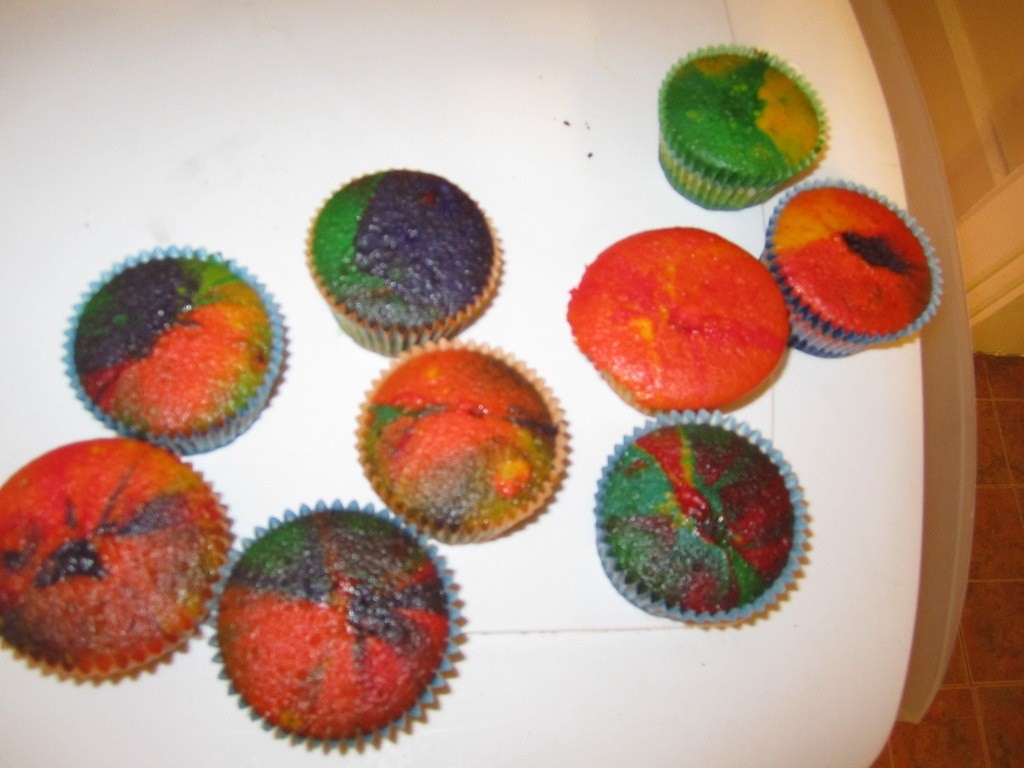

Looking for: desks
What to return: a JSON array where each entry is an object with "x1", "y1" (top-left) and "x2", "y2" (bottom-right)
[{"x1": 0, "y1": 1, "x2": 927, "y2": 768}]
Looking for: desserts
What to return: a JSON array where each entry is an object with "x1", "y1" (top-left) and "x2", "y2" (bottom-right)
[
  {"x1": 593, "y1": 407, "x2": 809, "y2": 624},
  {"x1": 567, "y1": 42, "x2": 947, "y2": 420},
  {"x1": 0, "y1": 169, "x2": 570, "y2": 750}
]
